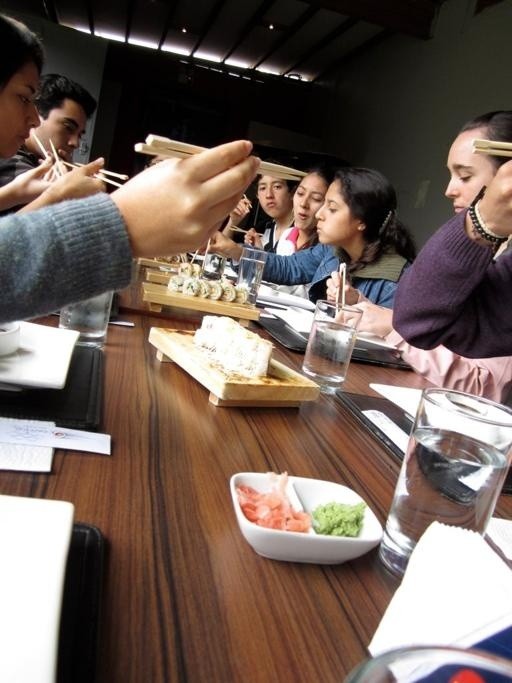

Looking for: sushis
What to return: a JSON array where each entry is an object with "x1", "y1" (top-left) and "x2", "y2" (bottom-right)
[{"x1": 166, "y1": 262, "x2": 249, "y2": 304}]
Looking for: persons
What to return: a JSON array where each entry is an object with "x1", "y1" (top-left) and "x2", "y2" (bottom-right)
[
  {"x1": 196, "y1": 109, "x2": 511, "y2": 407},
  {"x1": 0, "y1": 14, "x2": 263, "y2": 324}
]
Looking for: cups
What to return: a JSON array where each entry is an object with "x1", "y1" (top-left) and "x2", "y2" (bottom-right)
[
  {"x1": 378, "y1": 387, "x2": 512, "y2": 583},
  {"x1": 236, "y1": 247, "x2": 269, "y2": 306},
  {"x1": 298, "y1": 299, "x2": 364, "y2": 395},
  {"x1": 58, "y1": 290, "x2": 113, "y2": 346}
]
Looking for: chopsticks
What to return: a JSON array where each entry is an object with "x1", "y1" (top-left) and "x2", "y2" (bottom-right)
[
  {"x1": 32, "y1": 133, "x2": 310, "y2": 188},
  {"x1": 190, "y1": 238, "x2": 211, "y2": 271},
  {"x1": 472, "y1": 139, "x2": 512, "y2": 158},
  {"x1": 242, "y1": 192, "x2": 252, "y2": 209},
  {"x1": 228, "y1": 224, "x2": 266, "y2": 237},
  {"x1": 334, "y1": 263, "x2": 347, "y2": 320}
]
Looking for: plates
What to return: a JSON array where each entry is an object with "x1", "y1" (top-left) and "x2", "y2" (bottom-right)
[
  {"x1": 229, "y1": 471, "x2": 383, "y2": 566},
  {"x1": 239, "y1": 280, "x2": 400, "y2": 353},
  {"x1": 0, "y1": 321, "x2": 80, "y2": 390}
]
